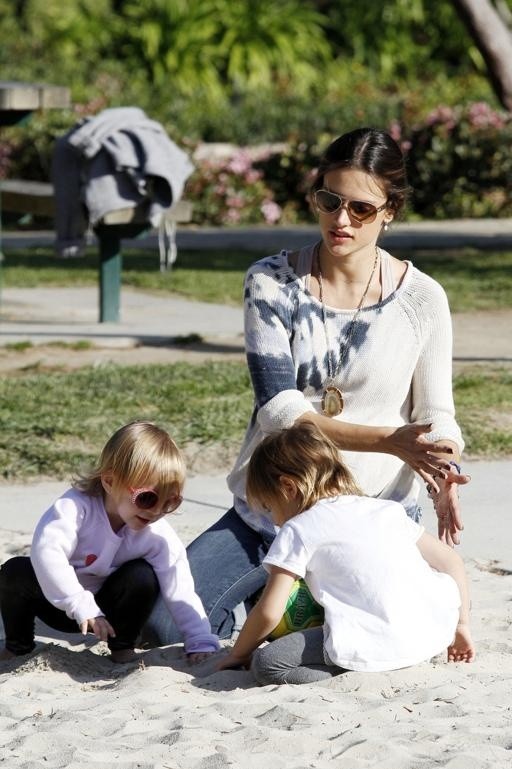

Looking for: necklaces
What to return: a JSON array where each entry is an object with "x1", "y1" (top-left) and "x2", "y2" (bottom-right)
[{"x1": 316, "y1": 236, "x2": 378, "y2": 417}]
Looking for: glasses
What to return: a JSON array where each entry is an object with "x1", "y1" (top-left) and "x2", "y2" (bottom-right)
[
  {"x1": 126, "y1": 481, "x2": 186, "y2": 516},
  {"x1": 308, "y1": 186, "x2": 393, "y2": 227}
]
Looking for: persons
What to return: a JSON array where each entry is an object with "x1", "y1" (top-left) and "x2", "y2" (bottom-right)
[
  {"x1": 139, "y1": 126, "x2": 472, "y2": 648},
  {"x1": 195, "y1": 418, "x2": 476, "y2": 686},
  {"x1": 1, "y1": 420, "x2": 221, "y2": 666}
]
